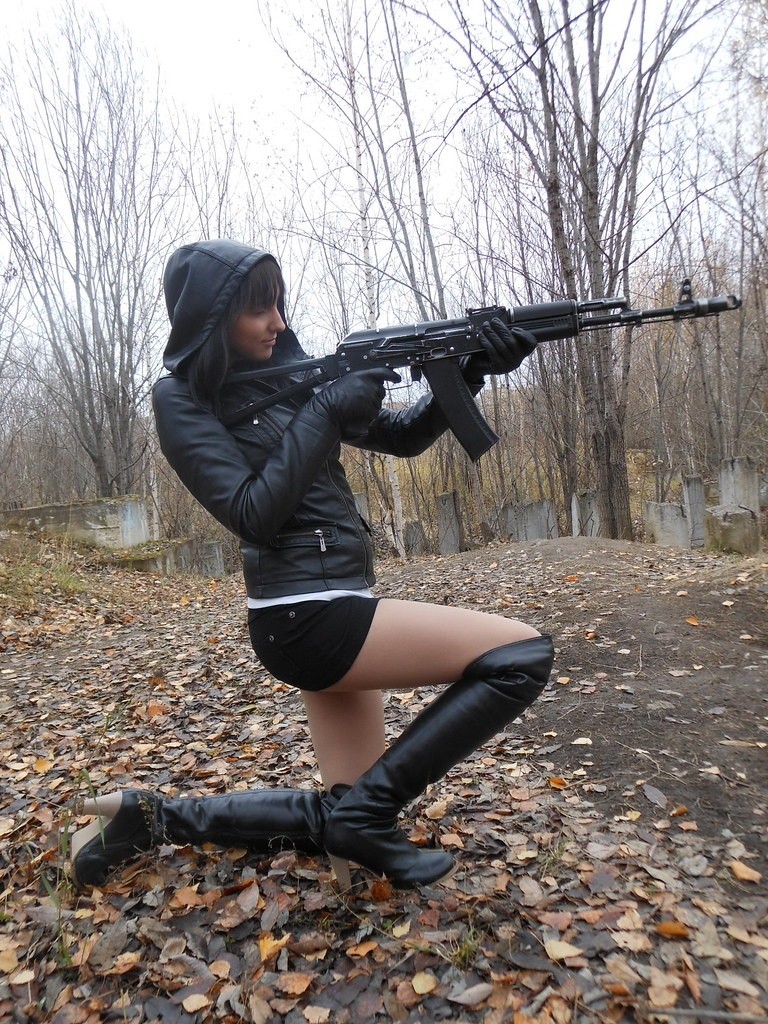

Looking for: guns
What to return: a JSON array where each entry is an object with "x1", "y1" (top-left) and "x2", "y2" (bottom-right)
[{"x1": 213, "y1": 278, "x2": 746, "y2": 461}]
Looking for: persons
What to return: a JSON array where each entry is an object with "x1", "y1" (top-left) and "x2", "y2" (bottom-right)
[{"x1": 72, "y1": 238, "x2": 555, "y2": 894}]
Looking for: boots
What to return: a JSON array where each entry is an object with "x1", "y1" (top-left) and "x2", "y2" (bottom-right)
[
  {"x1": 324, "y1": 632, "x2": 555, "y2": 894},
  {"x1": 70, "y1": 782, "x2": 354, "y2": 893}
]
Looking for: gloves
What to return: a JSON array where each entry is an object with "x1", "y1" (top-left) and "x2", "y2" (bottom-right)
[
  {"x1": 464, "y1": 317, "x2": 537, "y2": 383},
  {"x1": 304, "y1": 367, "x2": 401, "y2": 428}
]
